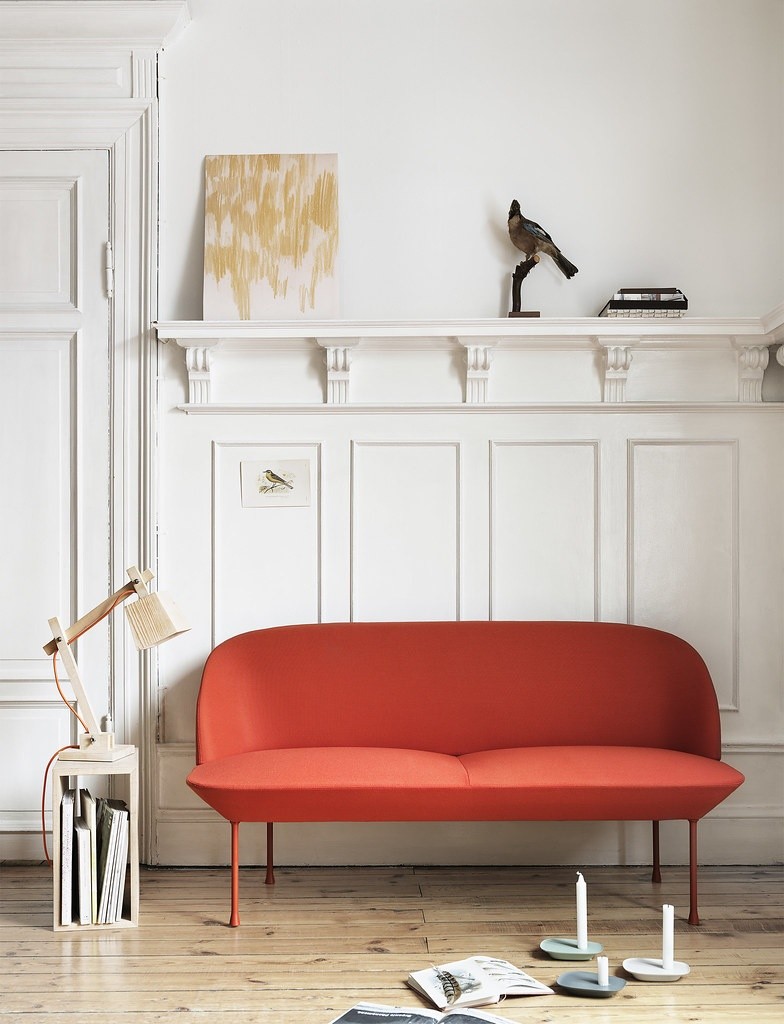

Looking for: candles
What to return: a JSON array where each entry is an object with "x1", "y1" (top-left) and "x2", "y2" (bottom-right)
[
  {"x1": 575, "y1": 872, "x2": 588, "y2": 949},
  {"x1": 662, "y1": 903, "x2": 675, "y2": 970},
  {"x1": 597, "y1": 956, "x2": 610, "y2": 986}
]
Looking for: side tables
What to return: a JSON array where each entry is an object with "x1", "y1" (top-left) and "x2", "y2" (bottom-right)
[{"x1": 51, "y1": 748, "x2": 142, "y2": 933}]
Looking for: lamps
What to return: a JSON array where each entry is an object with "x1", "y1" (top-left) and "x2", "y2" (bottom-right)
[{"x1": 42, "y1": 565, "x2": 192, "y2": 763}]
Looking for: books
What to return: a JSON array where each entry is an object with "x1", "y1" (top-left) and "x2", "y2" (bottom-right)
[
  {"x1": 404, "y1": 955, "x2": 556, "y2": 1012},
  {"x1": 328, "y1": 1001, "x2": 518, "y2": 1024},
  {"x1": 59, "y1": 789, "x2": 130, "y2": 926},
  {"x1": 598, "y1": 287, "x2": 688, "y2": 318}
]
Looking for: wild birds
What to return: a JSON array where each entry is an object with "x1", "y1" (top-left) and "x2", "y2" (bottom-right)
[{"x1": 507, "y1": 199, "x2": 578, "y2": 280}]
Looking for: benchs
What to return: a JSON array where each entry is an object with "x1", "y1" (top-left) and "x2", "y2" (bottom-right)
[{"x1": 185, "y1": 621, "x2": 744, "y2": 926}]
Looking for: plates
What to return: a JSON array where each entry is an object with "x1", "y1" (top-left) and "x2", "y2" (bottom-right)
[
  {"x1": 540, "y1": 939, "x2": 603, "y2": 961},
  {"x1": 557, "y1": 971, "x2": 626, "y2": 998},
  {"x1": 622, "y1": 957, "x2": 691, "y2": 982}
]
